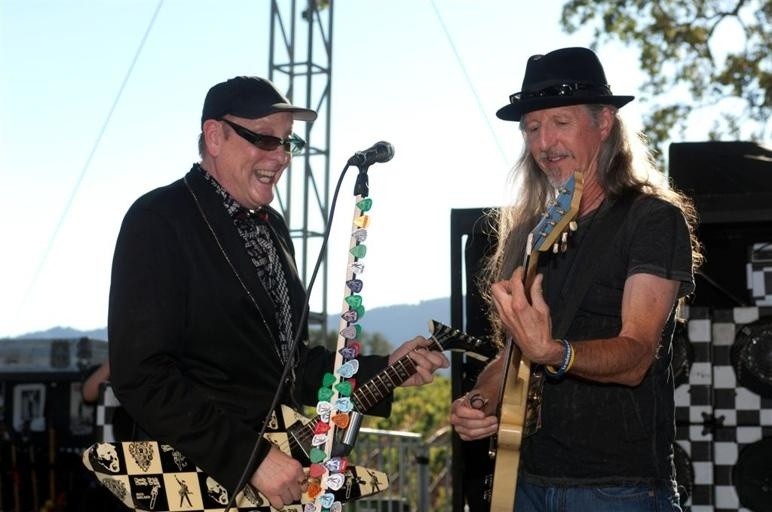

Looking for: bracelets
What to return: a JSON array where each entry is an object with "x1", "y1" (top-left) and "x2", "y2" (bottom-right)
[{"x1": 545, "y1": 335, "x2": 574, "y2": 377}]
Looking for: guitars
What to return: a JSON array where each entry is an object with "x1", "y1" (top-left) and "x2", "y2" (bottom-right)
[
  {"x1": 81, "y1": 320, "x2": 500, "y2": 510},
  {"x1": 462, "y1": 170, "x2": 584, "y2": 511}
]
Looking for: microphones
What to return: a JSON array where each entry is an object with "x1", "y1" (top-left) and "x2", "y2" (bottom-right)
[{"x1": 347, "y1": 141, "x2": 393, "y2": 166}]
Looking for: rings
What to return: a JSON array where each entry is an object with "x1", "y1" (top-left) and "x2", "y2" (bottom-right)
[{"x1": 298, "y1": 477, "x2": 308, "y2": 485}]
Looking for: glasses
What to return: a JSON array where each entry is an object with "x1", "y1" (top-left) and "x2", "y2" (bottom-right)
[
  {"x1": 509, "y1": 80, "x2": 606, "y2": 105},
  {"x1": 221, "y1": 118, "x2": 306, "y2": 156}
]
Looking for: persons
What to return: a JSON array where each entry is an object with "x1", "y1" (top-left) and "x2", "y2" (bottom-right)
[
  {"x1": 174, "y1": 475, "x2": 193, "y2": 508},
  {"x1": 82, "y1": 361, "x2": 112, "y2": 406},
  {"x1": 366, "y1": 470, "x2": 381, "y2": 494},
  {"x1": 450, "y1": 48, "x2": 702, "y2": 511},
  {"x1": 106, "y1": 76, "x2": 450, "y2": 511}
]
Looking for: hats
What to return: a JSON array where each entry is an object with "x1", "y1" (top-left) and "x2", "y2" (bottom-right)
[
  {"x1": 495, "y1": 46, "x2": 635, "y2": 123},
  {"x1": 200, "y1": 75, "x2": 318, "y2": 122}
]
